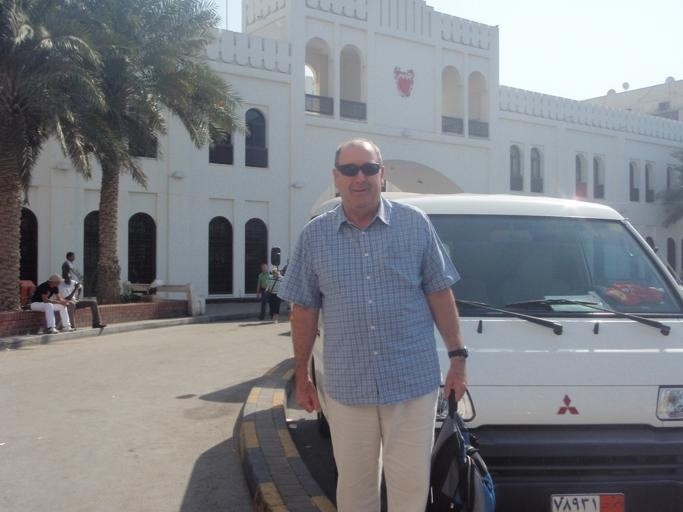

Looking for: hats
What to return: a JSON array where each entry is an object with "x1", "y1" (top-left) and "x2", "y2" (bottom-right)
[{"x1": 47, "y1": 274, "x2": 65, "y2": 282}]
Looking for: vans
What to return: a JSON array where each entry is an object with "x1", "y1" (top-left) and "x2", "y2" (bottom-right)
[{"x1": 305, "y1": 188, "x2": 683, "y2": 511}]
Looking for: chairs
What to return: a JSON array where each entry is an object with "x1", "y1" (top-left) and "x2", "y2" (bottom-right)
[{"x1": 499, "y1": 249, "x2": 573, "y2": 303}]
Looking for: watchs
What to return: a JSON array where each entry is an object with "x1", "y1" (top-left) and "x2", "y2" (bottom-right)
[{"x1": 446, "y1": 348, "x2": 468, "y2": 359}]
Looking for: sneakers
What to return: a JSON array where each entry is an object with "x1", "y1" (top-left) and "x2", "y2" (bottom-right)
[{"x1": 47, "y1": 327, "x2": 76, "y2": 333}]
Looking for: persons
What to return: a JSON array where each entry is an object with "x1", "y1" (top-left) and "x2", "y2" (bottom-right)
[
  {"x1": 276, "y1": 138, "x2": 469, "y2": 512},
  {"x1": 28, "y1": 251, "x2": 107, "y2": 335},
  {"x1": 256, "y1": 262, "x2": 282, "y2": 322}
]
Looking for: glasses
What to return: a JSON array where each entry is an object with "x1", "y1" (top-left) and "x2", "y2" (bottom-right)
[{"x1": 336, "y1": 163, "x2": 381, "y2": 176}]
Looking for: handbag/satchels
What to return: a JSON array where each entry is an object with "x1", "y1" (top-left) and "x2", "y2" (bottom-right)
[{"x1": 265, "y1": 294, "x2": 272, "y2": 302}]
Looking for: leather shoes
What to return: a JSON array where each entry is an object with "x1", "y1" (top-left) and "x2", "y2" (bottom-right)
[{"x1": 93, "y1": 323, "x2": 106, "y2": 328}]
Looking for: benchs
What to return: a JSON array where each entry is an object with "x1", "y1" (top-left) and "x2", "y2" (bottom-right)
[{"x1": 125, "y1": 281, "x2": 206, "y2": 317}]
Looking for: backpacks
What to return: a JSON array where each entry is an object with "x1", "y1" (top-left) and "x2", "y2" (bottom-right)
[{"x1": 425, "y1": 389, "x2": 496, "y2": 512}]
